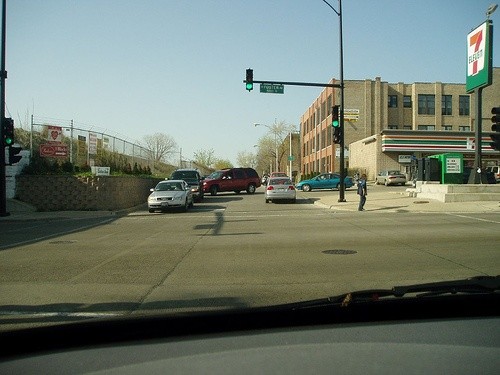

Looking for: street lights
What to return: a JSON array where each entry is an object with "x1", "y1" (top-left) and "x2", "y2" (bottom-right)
[
  {"x1": 254, "y1": 144, "x2": 273, "y2": 176},
  {"x1": 255, "y1": 123, "x2": 278, "y2": 173}
]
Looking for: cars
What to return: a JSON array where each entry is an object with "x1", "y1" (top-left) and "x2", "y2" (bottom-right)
[
  {"x1": 147, "y1": 180, "x2": 194, "y2": 213},
  {"x1": 264, "y1": 176, "x2": 297, "y2": 203},
  {"x1": 266, "y1": 172, "x2": 289, "y2": 186},
  {"x1": 295, "y1": 173, "x2": 356, "y2": 191},
  {"x1": 376, "y1": 169, "x2": 407, "y2": 186}
]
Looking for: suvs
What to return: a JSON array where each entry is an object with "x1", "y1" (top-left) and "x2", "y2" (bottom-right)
[{"x1": 164, "y1": 169, "x2": 205, "y2": 203}]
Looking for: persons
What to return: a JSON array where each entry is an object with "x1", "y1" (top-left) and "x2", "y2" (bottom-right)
[{"x1": 357, "y1": 174, "x2": 367, "y2": 211}]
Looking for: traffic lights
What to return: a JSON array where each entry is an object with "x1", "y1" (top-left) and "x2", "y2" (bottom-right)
[
  {"x1": 489, "y1": 106, "x2": 500, "y2": 151},
  {"x1": 4, "y1": 117, "x2": 15, "y2": 146},
  {"x1": 331, "y1": 104, "x2": 339, "y2": 127},
  {"x1": 9, "y1": 146, "x2": 23, "y2": 162},
  {"x1": 245, "y1": 68, "x2": 253, "y2": 90},
  {"x1": 333, "y1": 127, "x2": 341, "y2": 143}
]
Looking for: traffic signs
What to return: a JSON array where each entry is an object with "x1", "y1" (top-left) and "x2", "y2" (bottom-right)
[{"x1": 40, "y1": 143, "x2": 68, "y2": 155}]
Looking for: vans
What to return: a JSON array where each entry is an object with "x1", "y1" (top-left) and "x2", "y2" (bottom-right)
[
  {"x1": 483, "y1": 166, "x2": 500, "y2": 183},
  {"x1": 202, "y1": 168, "x2": 261, "y2": 196}
]
